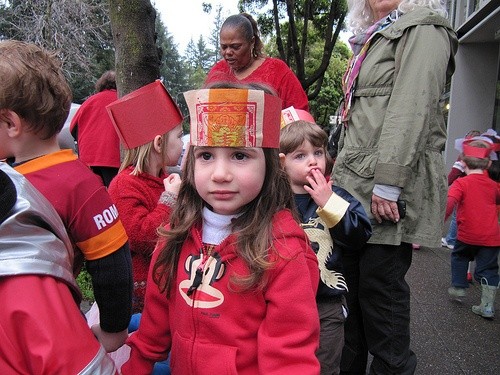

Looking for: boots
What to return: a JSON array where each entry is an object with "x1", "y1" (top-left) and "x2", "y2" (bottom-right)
[
  {"x1": 448, "y1": 286, "x2": 466, "y2": 302},
  {"x1": 471, "y1": 277, "x2": 500, "y2": 318}
]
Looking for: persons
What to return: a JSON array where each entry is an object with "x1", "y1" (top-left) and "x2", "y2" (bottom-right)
[
  {"x1": 0, "y1": 35, "x2": 500, "y2": 375},
  {"x1": 328, "y1": 0, "x2": 457, "y2": 375},
  {"x1": 200, "y1": 14, "x2": 310, "y2": 115}
]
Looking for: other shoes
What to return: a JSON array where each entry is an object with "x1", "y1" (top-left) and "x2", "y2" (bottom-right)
[{"x1": 441, "y1": 238, "x2": 454, "y2": 249}]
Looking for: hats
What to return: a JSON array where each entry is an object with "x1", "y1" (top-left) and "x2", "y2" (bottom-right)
[
  {"x1": 105, "y1": 80, "x2": 183, "y2": 150},
  {"x1": 281, "y1": 106, "x2": 317, "y2": 130}
]
